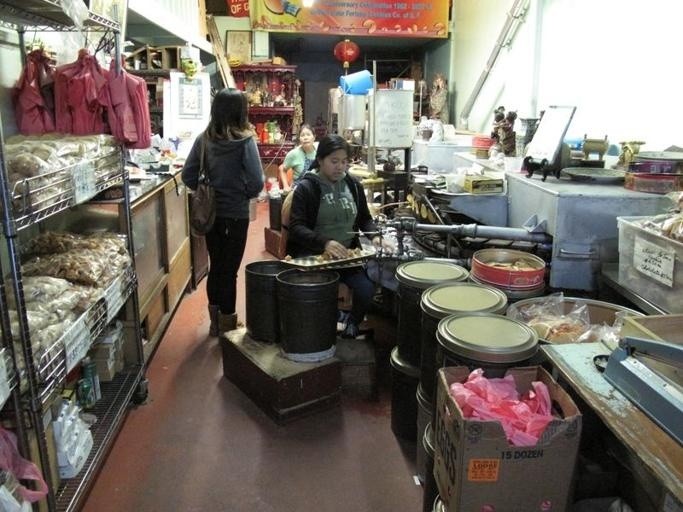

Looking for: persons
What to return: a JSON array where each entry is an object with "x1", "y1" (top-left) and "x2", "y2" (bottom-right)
[
  {"x1": 429, "y1": 74, "x2": 450, "y2": 123},
  {"x1": 278, "y1": 124, "x2": 319, "y2": 192},
  {"x1": 284, "y1": 134, "x2": 396, "y2": 340},
  {"x1": 181, "y1": 87, "x2": 263, "y2": 339}
]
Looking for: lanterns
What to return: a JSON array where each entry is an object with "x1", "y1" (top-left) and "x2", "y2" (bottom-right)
[{"x1": 333, "y1": 39, "x2": 360, "y2": 69}]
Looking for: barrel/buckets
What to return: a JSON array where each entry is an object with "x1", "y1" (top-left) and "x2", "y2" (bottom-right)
[
  {"x1": 339, "y1": 69, "x2": 373, "y2": 94},
  {"x1": 269, "y1": 195, "x2": 287, "y2": 233},
  {"x1": 276, "y1": 269, "x2": 340, "y2": 362},
  {"x1": 389, "y1": 249, "x2": 649, "y2": 511},
  {"x1": 245, "y1": 261, "x2": 287, "y2": 342}
]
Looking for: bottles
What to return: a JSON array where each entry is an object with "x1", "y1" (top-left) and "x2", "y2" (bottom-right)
[{"x1": 83, "y1": 361, "x2": 101, "y2": 400}]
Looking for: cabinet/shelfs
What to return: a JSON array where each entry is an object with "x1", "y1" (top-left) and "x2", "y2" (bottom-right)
[
  {"x1": 230, "y1": 64, "x2": 298, "y2": 165},
  {"x1": 80, "y1": 161, "x2": 192, "y2": 368},
  {"x1": 188, "y1": 193, "x2": 209, "y2": 290}
]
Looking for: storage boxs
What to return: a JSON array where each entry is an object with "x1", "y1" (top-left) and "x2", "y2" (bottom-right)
[
  {"x1": 432, "y1": 364, "x2": 583, "y2": 512},
  {"x1": 125, "y1": 45, "x2": 200, "y2": 108},
  {"x1": 540, "y1": 341, "x2": 683, "y2": 511},
  {"x1": 616, "y1": 214, "x2": 683, "y2": 315}
]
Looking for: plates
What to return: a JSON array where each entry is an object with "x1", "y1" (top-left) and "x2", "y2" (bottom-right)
[{"x1": 562, "y1": 166, "x2": 628, "y2": 182}]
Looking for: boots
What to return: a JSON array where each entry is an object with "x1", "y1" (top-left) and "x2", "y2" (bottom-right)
[{"x1": 208, "y1": 305, "x2": 245, "y2": 339}]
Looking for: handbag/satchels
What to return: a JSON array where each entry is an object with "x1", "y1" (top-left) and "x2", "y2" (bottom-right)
[{"x1": 188, "y1": 170, "x2": 216, "y2": 236}]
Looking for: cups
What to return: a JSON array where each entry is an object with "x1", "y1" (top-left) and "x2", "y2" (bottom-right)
[{"x1": 420, "y1": 130, "x2": 431, "y2": 140}]
